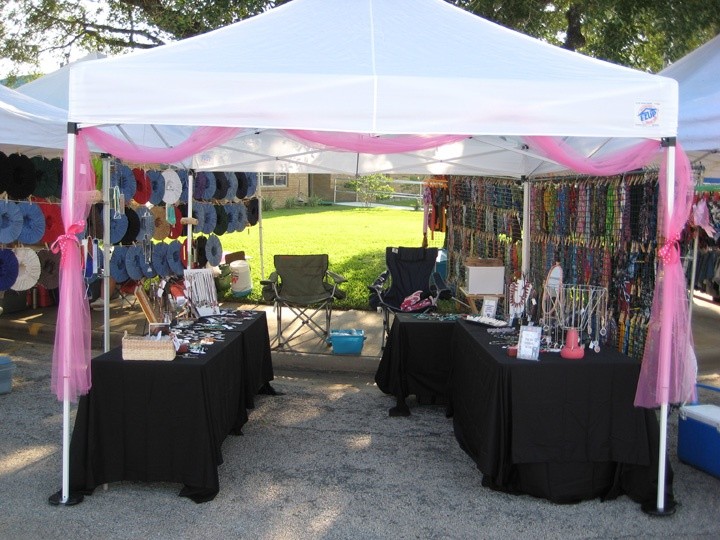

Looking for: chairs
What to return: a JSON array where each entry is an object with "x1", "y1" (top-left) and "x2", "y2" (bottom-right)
[
  {"x1": 258, "y1": 255, "x2": 349, "y2": 354},
  {"x1": 368, "y1": 244, "x2": 457, "y2": 353}
]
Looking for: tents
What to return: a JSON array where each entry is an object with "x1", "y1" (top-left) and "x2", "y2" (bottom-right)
[
  {"x1": 654, "y1": 32, "x2": 720, "y2": 346},
  {"x1": 52, "y1": 1, "x2": 691, "y2": 516},
  {"x1": 0, "y1": 49, "x2": 141, "y2": 354}
]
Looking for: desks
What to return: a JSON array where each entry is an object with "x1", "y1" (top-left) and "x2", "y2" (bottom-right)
[
  {"x1": 373, "y1": 311, "x2": 674, "y2": 516},
  {"x1": 66, "y1": 309, "x2": 278, "y2": 505}
]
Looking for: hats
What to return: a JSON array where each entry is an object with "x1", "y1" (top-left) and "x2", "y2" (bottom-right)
[
  {"x1": 0, "y1": 198, "x2": 65, "y2": 244},
  {"x1": 0, "y1": 247, "x2": 59, "y2": 290},
  {"x1": 86, "y1": 156, "x2": 260, "y2": 284},
  {"x1": 0, "y1": 151, "x2": 64, "y2": 198}
]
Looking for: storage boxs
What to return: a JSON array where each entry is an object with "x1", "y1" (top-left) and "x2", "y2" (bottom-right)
[
  {"x1": 676, "y1": 405, "x2": 720, "y2": 479},
  {"x1": 464, "y1": 257, "x2": 506, "y2": 296}
]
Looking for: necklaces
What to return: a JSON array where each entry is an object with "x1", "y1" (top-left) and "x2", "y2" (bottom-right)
[{"x1": 540, "y1": 286, "x2": 610, "y2": 353}]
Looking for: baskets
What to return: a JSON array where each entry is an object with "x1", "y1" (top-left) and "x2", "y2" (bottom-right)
[{"x1": 121, "y1": 335, "x2": 177, "y2": 360}]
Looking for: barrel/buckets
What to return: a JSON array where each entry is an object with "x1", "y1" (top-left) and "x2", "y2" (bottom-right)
[
  {"x1": 0, "y1": 356, "x2": 13, "y2": 394},
  {"x1": 230, "y1": 260, "x2": 253, "y2": 292}
]
